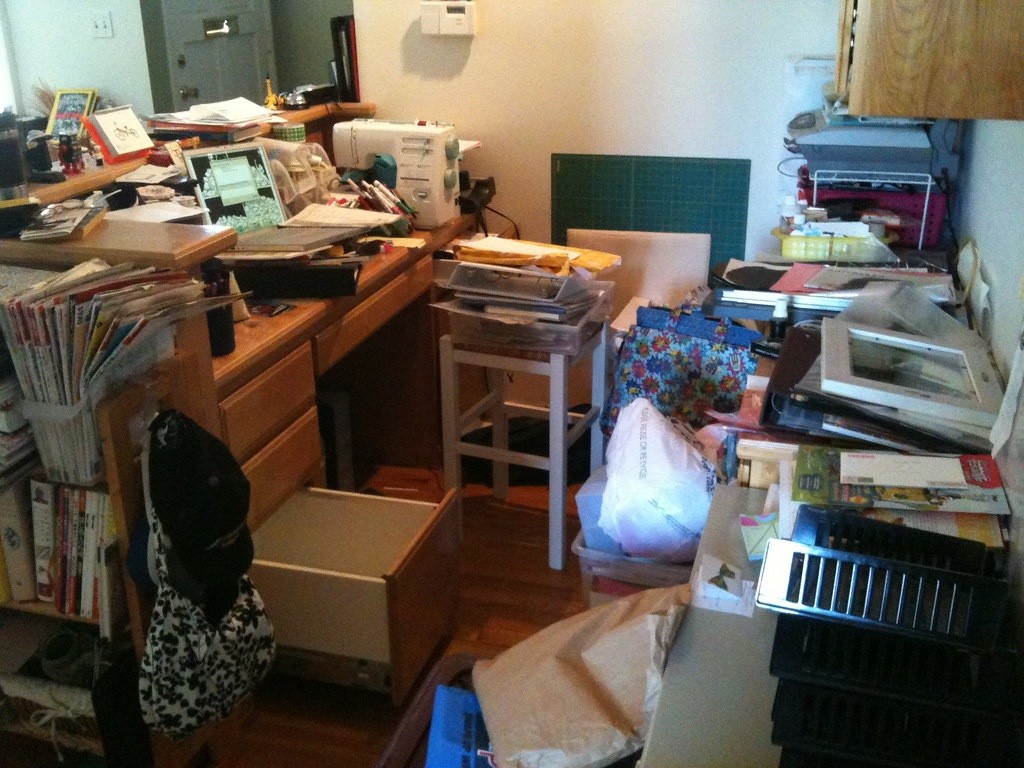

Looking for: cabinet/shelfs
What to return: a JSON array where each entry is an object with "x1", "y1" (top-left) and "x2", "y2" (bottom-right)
[
  {"x1": 829, "y1": 0, "x2": 1024, "y2": 119},
  {"x1": 0, "y1": 105, "x2": 479, "y2": 768}
]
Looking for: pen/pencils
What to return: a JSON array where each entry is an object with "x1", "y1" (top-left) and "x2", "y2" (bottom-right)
[{"x1": 345, "y1": 178, "x2": 417, "y2": 234}]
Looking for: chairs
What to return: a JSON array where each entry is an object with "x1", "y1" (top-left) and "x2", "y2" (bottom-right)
[{"x1": 567, "y1": 230, "x2": 714, "y2": 330}]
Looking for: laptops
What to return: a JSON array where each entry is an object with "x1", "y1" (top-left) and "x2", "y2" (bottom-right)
[{"x1": 180, "y1": 142, "x2": 372, "y2": 251}]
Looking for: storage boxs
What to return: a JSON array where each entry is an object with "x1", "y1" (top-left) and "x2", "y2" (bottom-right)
[
  {"x1": 574, "y1": 465, "x2": 693, "y2": 613},
  {"x1": 797, "y1": 165, "x2": 945, "y2": 251},
  {"x1": 385, "y1": 654, "x2": 493, "y2": 768},
  {"x1": 18, "y1": 330, "x2": 177, "y2": 483},
  {"x1": 449, "y1": 279, "x2": 618, "y2": 357}
]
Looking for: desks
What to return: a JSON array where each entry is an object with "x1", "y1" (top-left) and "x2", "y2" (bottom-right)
[{"x1": 638, "y1": 325, "x2": 1024, "y2": 766}]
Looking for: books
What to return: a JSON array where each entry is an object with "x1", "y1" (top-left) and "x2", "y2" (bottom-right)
[
  {"x1": 455, "y1": 289, "x2": 597, "y2": 322},
  {"x1": 20, "y1": 203, "x2": 108, "y2": 241},
  {"x1": 736, "y1": 388, "x2": 1012, "y2": 549},
  {"x1": 721, "y1": 259, "x2": 956, "y2": 312},
  {"x1": 0, "y1": 256, "x2": 252, "y2": 642},
  {"x1": 279, "y1": 202, "x2": 400, "y2": 228}
]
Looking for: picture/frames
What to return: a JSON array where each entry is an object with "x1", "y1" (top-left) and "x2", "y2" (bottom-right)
[{"x1": 821, "y1": 316, "x2": 1003, "y2": 427}]
